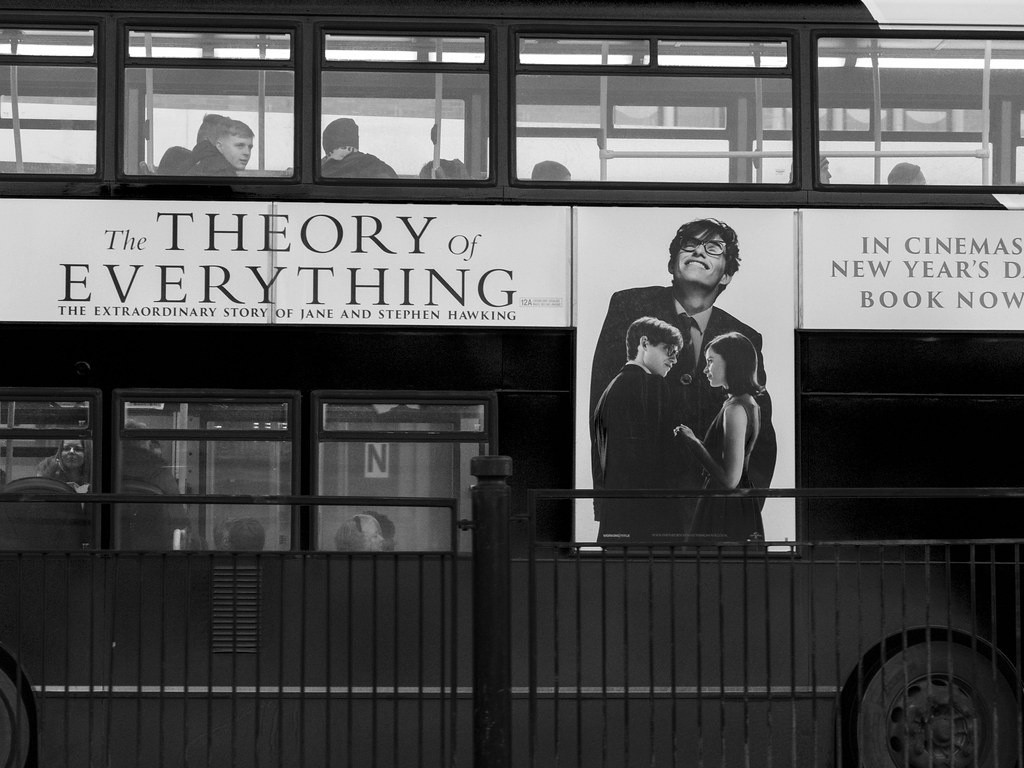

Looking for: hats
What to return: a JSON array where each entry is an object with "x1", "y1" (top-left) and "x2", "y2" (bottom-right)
[
  {"x1": 888, "y1": 163, "x2": 926, "y2": 184},
  {"x1": 790, "y1": 156, "x2": 828, "y2": 181},
  {"x1": 531, "y1": 161, "x2": 569, "y2": 180},
  {"x1": 323, "y1": 118, "x2": 359, "y2": 154},
  {"x1": 230, "y1": 518, "x2": 264, "y2": 549}
]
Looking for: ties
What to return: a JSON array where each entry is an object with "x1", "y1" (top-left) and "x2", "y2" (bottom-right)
[{"x1": 678, "y1": 313, "x2": 696, "y2": 373}]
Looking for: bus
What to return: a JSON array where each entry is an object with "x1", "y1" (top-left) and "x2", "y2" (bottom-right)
[{"x1": 0, "y1": 1, "x2": 1024, "y2": 768}]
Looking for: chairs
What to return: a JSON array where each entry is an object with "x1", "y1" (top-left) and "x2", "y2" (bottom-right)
[
  {"x1": 83, "y1": 469, "x2": 181, "y2": 552},
  {"x1": 139, "y1": 161, "x2": 152, "y2": 174},
  {"x1": 0, "y1": 477, "x2": 84, "y2": 553}
]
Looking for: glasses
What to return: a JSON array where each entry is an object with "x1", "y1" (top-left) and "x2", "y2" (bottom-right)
[
  {"x1": 681, "y1": 237, "x2": 727, "y2": 259},
  {"x1": 659, "y1": 343, "x2": 680, "y2": 359}
]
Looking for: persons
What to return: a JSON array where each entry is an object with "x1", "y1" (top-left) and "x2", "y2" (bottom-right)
[
  {"x1": 122, "y1": 419, "x2": 190, "y2": 532},
  {"x1": 887, "y1": 162, "x2": 927, "y2": 185},
  {"x1": 155, "y1": 114, "x2": 254, "y2": 177},
  {"x1": 321, "y1": 118, "x2": 398, "y2": 179},
  {"x1": 36, "y1": 434, "x2": 90, "y2": 502},
  {"x1": 531, "y1": 160, "x2": 571, "y2": 181},
  {"x1": 589, "y1": 218, "x2": 778, "y2": 540},
  {"x1": 593, "y1": 316, "x2": 684, "y2": 546},
  {"x1": 820, "y1": 154, "x2": 832, "y2": 183},
  {"x1": 213, "y1": 517, "x2": 264, "y2": 554},
  {"x1": 672, "y1": 332, "x2": 768, "y2": 555},
  {"x1": 331, "y1": 511, "x2": 398, "y2": 556}
]
